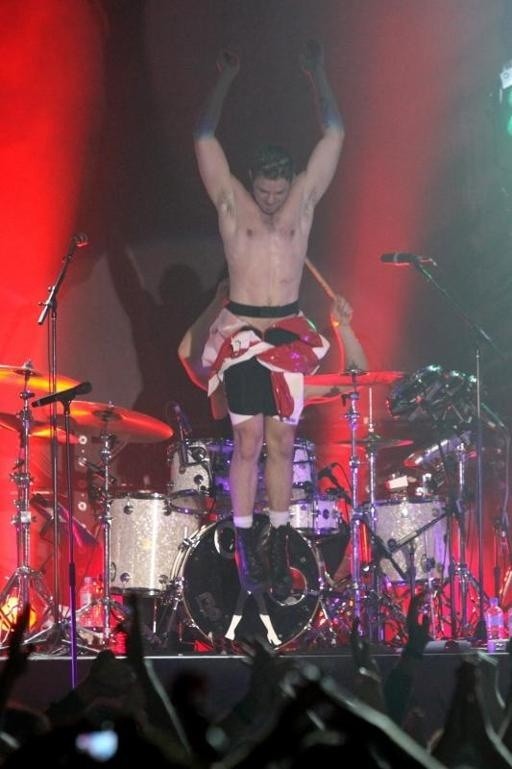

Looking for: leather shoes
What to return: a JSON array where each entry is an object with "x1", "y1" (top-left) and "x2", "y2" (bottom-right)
[
  {"x1": 233, "y1": 524, "x2": 269, "y2": 594},
  {"x1": 257, "y1": 524, "x2": 294, "y2": 601}
]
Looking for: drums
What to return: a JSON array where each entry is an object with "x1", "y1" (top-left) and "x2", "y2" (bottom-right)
[
  {"x1": 361, "y1": 495, "x2": 452, "y2": 586},
  {"x1": 253, "y1": 434, "x2": 318, "y2": 508},
  {"x1": 108, "y1": 487, "x2": 202, "y2": 599},
  {"x1": 154, "y1": 508, "x2": 334, "y2": 655},
  {"x1": 162, "y1": 435, "x2": 235, "y2": 517},
  {"x1": 288, "y1": 496, "x2": 343, "y2": 537},
  {"x1": 403, "y1": 428, "x2": 502, "y2": 507}
]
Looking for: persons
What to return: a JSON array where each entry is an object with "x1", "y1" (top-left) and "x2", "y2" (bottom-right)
[
  {"x1": 1, "y1": 601, "x2": 215, "y2": 769},
  {"x1": 384, "y1": 593, "x2": 503, "y2": 769},
  {"x1": 202, "y1": 663, "x2": 445, "y2": 767},
  {"x1": 194, "y1": 44, "x2": 344, "y2": 599},
  {"x1": 348, "y1": 619, "x2": 380, "y2": 705}
]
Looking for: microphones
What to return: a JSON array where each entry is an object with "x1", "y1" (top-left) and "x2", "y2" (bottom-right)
[
  {"x1": 380, "y1": 252, "x2": 435, "y2": 270},
  {"x1": 64, "y1": 232, "x2": 90, "y2": 250},
  {"x1": 32, "y1": 382, "x2": 92, "y2": 410}
]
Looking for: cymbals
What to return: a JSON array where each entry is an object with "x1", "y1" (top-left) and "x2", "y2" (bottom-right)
[
  {"x1": 303, "y1": 369, "x2": 408, "y2": 388},
  {"x1": 0, "y1": 363, "x2": 81, "y2": 394},
  {"x1": 56, "y1": 400, "x2": 174, "y2": 444},
  {"x1": 334, "y1": 432, "x2": 415, "y2": 451},
  {"x1": 1, "y1": 411, "x2": 79, "y2": 445}
]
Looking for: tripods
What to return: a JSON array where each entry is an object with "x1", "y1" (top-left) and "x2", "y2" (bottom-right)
[
  {"x1": 419, "y1": 272, "x2": 512, "y2": 654},
  {"x1": 10, "y1": 253, "x2": 116, "y2": 658},
  {"x1": 301, "y1": 386, "x2": 437, "y2": 654}
]
